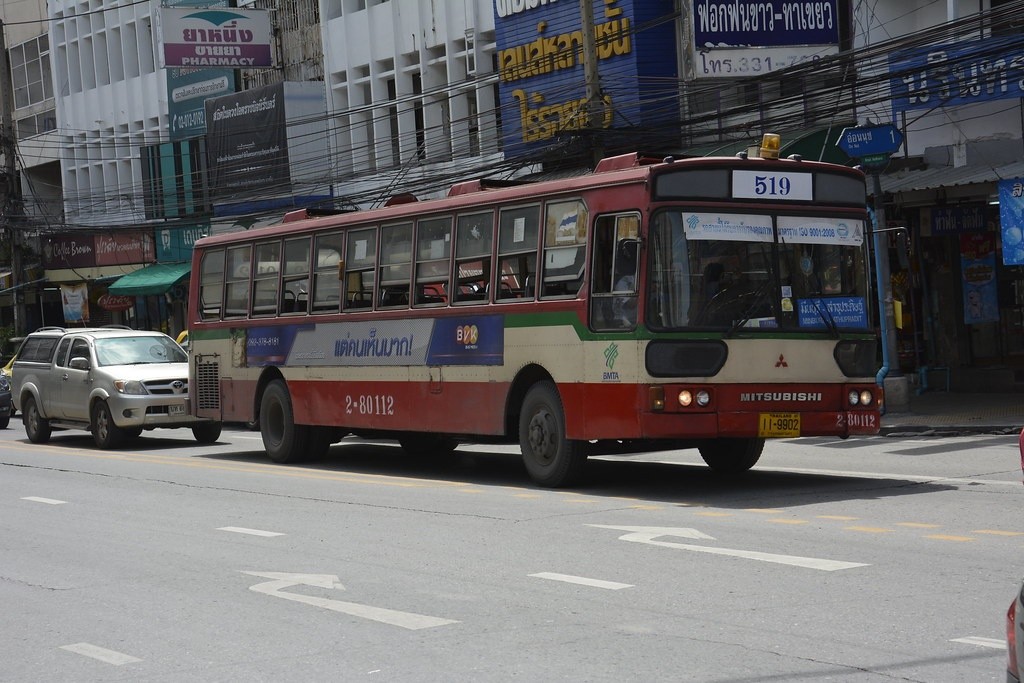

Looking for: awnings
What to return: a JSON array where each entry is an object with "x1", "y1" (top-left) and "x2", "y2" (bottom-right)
[
  {"x1": 108, "y1": 261, "x2": 192, "y2": 297},
  {"x1": 655, "y1": 121, "x2": 858, "y2": 166}
]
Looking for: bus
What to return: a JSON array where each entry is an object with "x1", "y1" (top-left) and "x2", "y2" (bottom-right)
[{"x1": 183, "y1": 131, "x2": 914, "y2": 490}]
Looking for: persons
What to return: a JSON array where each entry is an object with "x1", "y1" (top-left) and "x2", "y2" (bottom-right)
[{"x1": 612, "y1": 260, "x2": 736, "y2": 328}]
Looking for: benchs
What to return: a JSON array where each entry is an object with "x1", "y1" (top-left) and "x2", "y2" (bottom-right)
[{"x1": 244, "y1": 273, "x2": 548, "y2": 315}]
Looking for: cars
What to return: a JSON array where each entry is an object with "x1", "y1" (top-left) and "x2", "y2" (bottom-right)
[{"x1": 0, "y1": 368, "x2": 12, "y2": 429}]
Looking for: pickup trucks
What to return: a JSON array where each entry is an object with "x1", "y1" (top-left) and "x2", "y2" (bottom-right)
[{"x1": 10, "y1": 324, "x2": 223, "y2": 450}]
